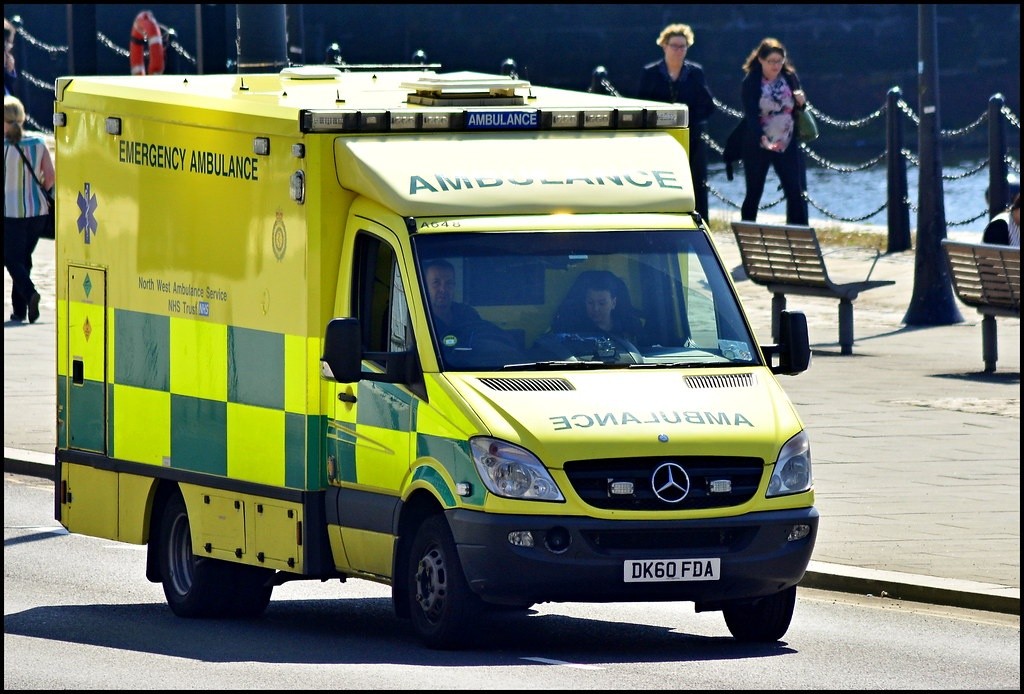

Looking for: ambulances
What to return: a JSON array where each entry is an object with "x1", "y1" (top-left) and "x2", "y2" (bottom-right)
[{"x1": 52, "y1": 67, "x2": 819, "y2": 648}]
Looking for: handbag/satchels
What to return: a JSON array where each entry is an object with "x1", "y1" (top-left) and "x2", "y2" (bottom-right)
[
  {"x1": 40, "y1": 189, "x2": 56, "y2": 239},
  {"x1": 796, "y1": 107, "x2": 819, "y2": 143}
]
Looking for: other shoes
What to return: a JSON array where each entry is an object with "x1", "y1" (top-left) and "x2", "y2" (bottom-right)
[
  {"x1": 10, "y1": 305, "x2": 26, "y2": 324},
  {"x1": 29, "y1": 291, "x2": 40, "y2": 324}
]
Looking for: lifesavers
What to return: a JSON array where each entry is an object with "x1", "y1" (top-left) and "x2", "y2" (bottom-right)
[{"x1": 128, "y1": 10, "x2": 164, "y2": 76}]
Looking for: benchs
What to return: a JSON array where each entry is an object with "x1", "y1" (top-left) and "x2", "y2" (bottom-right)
[
  {"x1": 941, "y1": 238, "x2": 1020, "y2": 374},
  {"x1": 730, "y1": 220, "x2": 896, "y2": 356}
]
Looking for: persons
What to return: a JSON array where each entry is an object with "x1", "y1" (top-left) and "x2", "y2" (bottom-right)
[
  {"x1": 4, "y1": 18, "x2": 18, "y2": 98},
  {"x1": 738, "y1": 37, "x2": 809, "y2": 227},
  {"x1": 982, "y1": 192, "x2": 1021, "y2": 248},
  {"x1": 422, "y1": 259, "x2": 484, "y2": 353},
  {"x1": 2, "y1": 94, "x2": 54, "y2": 325},
  {"x1": 639, "y1": 22, "x2": 717, "y2": 227},
  {"x1": 533, "y1": 269, "x2": 646, "y2": 350}
]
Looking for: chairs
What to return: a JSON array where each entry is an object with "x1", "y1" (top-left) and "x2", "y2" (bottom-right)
[{"x1": 553, "y1": 270, "x2": 637, "y2": 333}]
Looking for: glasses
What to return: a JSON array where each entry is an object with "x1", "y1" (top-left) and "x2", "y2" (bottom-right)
[
  {"x1": 765, "y1": 59, "x2": 783, "y2": 65},
  {"x1": 667, "y1": 44, "x2": 688, "y2": 50}
]
